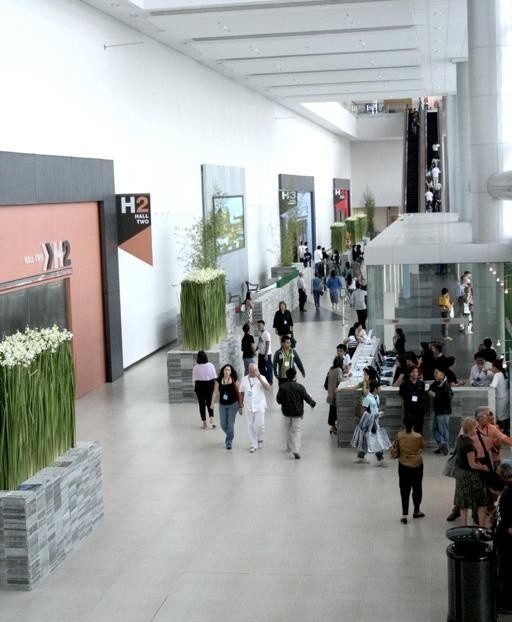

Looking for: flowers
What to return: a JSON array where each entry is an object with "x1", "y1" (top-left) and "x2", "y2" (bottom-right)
[
  {"x1": 317, "y1": 192, "x2": 381, "y2": 275},
  {"x1": 175, "y1": 264, "x2": 227, "y2": 351},
  {"x1": 0, "y1": 320, "x2": 76, "y2": 494}
]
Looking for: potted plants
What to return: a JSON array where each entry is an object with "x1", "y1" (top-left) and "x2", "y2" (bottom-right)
[{"x1": 268, "y1": 215, "x2": 305, "y2": 281}]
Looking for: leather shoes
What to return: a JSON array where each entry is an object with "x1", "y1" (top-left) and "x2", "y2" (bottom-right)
[
  {"x1": 414, "y1": 513, "x2": 425, "y2": 517},
  {"x1": 400, "y1": 518, "x2": 406, "y2": 524}
]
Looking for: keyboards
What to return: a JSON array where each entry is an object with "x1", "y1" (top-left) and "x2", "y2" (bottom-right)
[{"x1": 382, "y1": 362, "x2": 394, "y2": 385}]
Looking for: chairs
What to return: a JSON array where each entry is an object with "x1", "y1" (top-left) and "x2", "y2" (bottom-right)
[
  {"x1": 228, "y1": 293, "x2": 243, "y2": 325},
  {"x1": 245, "y1": 280, "x2": 260, "y2": 295}
]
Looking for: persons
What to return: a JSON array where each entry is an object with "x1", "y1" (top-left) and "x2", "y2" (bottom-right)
[
  {"x1": 210, "y1": 364, "x2": 242, "y2": 450},
  {"x1": 327, "y1": 270, "x2": 343, "y2": 308},
  {"x1": 396, "y1": 414, "x2": 426, "y2": 523},
  {"x1": 245, "y1": 292, "x2": 254, "y2": 323},
  {"x1": 297, "y1": 271, "x2": 308, "y2": 312},
  {"x1": 440, "y1": 264, "x2": 447, "y2": 275},
  {"x1": 298, "y1": 242, "x2": 340, "y2": 291},
  {"x1": 469, "y1": 338, "x2": 510, "y2": 437},
  {"x1": 351, "y1": 329, "x2": 457, "y2": 466},
  {"x1": 343, "y1": 261, "x2": 368, "y2": 329},
  {"x1": 457, "y1": 271, "x2": 474, "y2": 334},
  {"x1": 311, "y1": 272, "x2": 323, "y2": 310},
  {"x1": 326, "y1": 322, "x2": 367, "y2": 434},
  {"x1": 238, "y1": 300, "x2": 316, "y2": 459},
  {"x1": 455, "y1": 407, "x2": 512, "y2": 612},
  {"x1": 352, "y1": 244, "x2": 364, "y2": 263},
  {"x1": 408, "y1": 107, "x2": 442, "y2": 212},
  {"x1": 193, "y1": 351, "x2": 216, "y2": 428},
  {"x1": 438, "y1": 288, "x2": 454, "y2": 340}
]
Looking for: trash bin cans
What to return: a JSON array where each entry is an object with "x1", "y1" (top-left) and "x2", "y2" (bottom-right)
[{"x1": 445, "y1": 526, "x2": 498, "y2": 622}]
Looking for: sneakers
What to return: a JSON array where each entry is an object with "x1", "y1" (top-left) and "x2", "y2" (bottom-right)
[{"x1": 446, "y1": 511, "x2": 460, "y2": 521}]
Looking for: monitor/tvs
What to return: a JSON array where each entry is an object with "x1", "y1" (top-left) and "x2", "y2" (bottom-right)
[{"x1": 374, "y1": 344, "x2": 386, "y2": 380}]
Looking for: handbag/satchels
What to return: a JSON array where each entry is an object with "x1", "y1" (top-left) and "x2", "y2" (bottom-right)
[
  {"x1": 390, "y1": 440, "x2": 401, "y2": 459},
  {"x1": 463, "y1": 303, "x2": 471, "y2": 315},
  {"x1": 442, "y1": 451, "x2": 462, "y2": 478}
]
